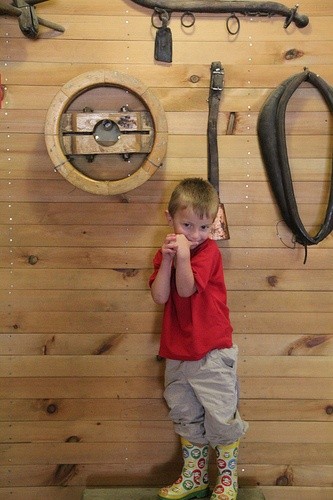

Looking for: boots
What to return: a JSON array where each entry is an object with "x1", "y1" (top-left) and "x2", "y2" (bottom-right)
[
  {"x1": 158, "y1": 437, "x2": 210, "y2": 500},
  {"x1": 211, "y1": 438, "x2": 240, "y2": 500}
]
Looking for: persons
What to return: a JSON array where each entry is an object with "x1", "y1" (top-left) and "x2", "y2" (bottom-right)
[{"x1": 149, "y1": 178, "x2": 248, "y2": 500}]
